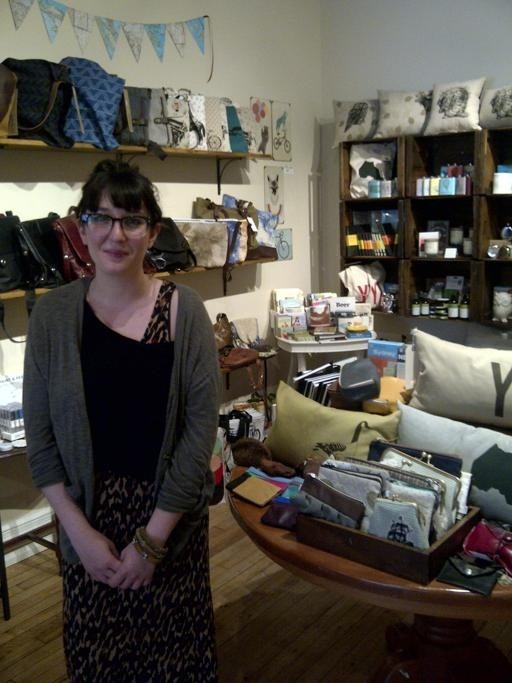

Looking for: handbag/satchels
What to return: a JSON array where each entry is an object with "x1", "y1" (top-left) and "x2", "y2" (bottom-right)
[
  {"x1": 260, "y1": 436, "x2": 511, "y2": 596},
  {"x1": 208, "y1": 391, "x2": 276, "y2": 506},
  {"x1": 114, "y1": 85, "x2": 262, "y2": 153},
  {"x1": 145, "y1": 194, "x2": 280, "y2": 271},
  {"x1": 337, "y1": 260, "x2": 387, "y2": 309},
  {"x1": 1, "y1": 57, "x2": 80, "y2": 149},
  {"x1": 59, "y1": 55, "x2": 125, "y2": 151},
  {"x1": 212, "y1": 312, "x2": 261, "y2": 369},
  {"x1": 0, "y1": 206, "x2": 97, "y2": 293},
  {"x1": 338, "y1": 357, "x2": 380, "y2": 401}
]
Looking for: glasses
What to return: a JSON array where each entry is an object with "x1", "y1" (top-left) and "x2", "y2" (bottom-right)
[{"x1": 77, "y1": 209, "x2": 154, "y2": 241}]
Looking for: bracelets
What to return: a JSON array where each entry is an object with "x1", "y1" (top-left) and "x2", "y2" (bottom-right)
[
  {"x1": 136, "y1": 526, "x2": 169, "y2": 559},
  {"x1": 130, "y1": 535, "x2": 162, "y2": 564}
]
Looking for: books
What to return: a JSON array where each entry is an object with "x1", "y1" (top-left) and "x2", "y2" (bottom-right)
[
  {"x1": 342, "y1": 221, "x2": 399, "y2": 258},
  {"x1": 290, "y1": 356, "x2": 361, "y2": 413},
  {"x1": 427, "y1": 219, "x2": 450, "y2": 254},
  {"x1": 272, "y1": 285, "x2": 377, "y2": 348},
  {"x1": 444, "y1": 275, "x2": 465, "y2": 302}
]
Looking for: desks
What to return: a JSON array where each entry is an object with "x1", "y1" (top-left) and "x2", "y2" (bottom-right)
[{"x1": 227, "y1": 464, "x2": 512, "y2": 682}]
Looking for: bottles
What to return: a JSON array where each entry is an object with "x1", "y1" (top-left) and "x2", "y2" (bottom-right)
[{"x1": 413, "y1": 293, "x2": 470, "y2": 320}]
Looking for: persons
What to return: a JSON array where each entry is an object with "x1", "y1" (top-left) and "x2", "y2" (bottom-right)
[{"x1": 21, "y1": 157, "x2": 228, "y2": 679}]
